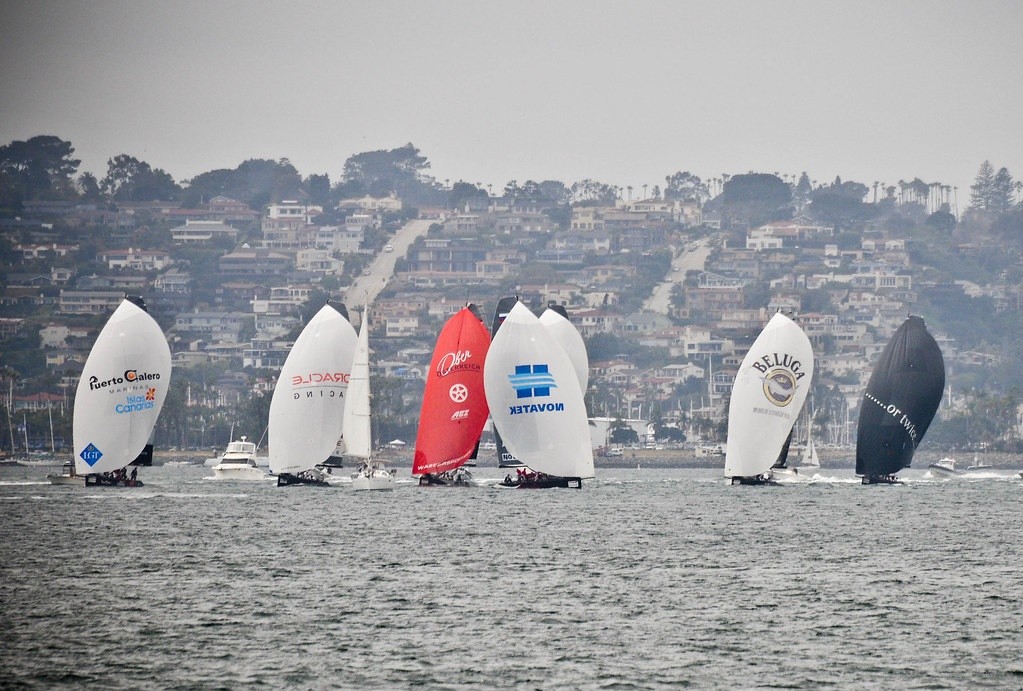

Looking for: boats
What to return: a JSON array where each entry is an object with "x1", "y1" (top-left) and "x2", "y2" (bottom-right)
[
  {"x1": 351, "y1": 459, "x2": 397, "y2": 491},
  {"x1": 46, "y1": 460, "x2": 96, "y2": 485},
  {"x1": 211, "y1": 422, "x2": 269, "y2": 481},
  {"x1": 966, "y1": 457, "x2": 991, "y2": 471},
  {"x1": 929, "y1": 457, "x2": 962, "y2": 480}
]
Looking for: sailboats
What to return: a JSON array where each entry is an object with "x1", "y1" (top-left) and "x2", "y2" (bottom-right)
[
  {"x1": 483, "y1": 297, "x2": 597, "y2": 489},
  {"x1": 723, "y1": 311, "x2": 820, "y2": 486},
  {"x1": 268, "y1": 300, "x2": 360, "y2": 488},
  {"x1": 855, "y1": 315, "x2": 945, "y2": 484},
  {"x1": 73, "y1": 294, "x2": 172, "y2": 487},
  {"x1": 0, "y1": 394, "x2": 66, "y2": 467},
  {"x1": 322, "y1": 303, "x2": 414, "y2": 487},
  {"x1": 411, "y1": 302, "x2": 492, "y2": 486}
]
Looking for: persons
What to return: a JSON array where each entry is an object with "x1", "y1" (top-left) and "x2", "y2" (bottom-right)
[
  {"x1": 297, "y1": 471, "x2": 314, "y2": 480},
  {"x1": 882, "y1": 473, "x2": 898, "y2": 481},
  {"x1": 504, "y1": 473, "x2": 512, "y2": 483},
  {"x1": 517, "y1": 468, "x2": 542, "y2": 481},
  {"x1": 104, "y1": 467, "x2": 137, "y2": 480},
  {"x1": 441, "y1": 472, "x2": 450, "y2": 480}
]
